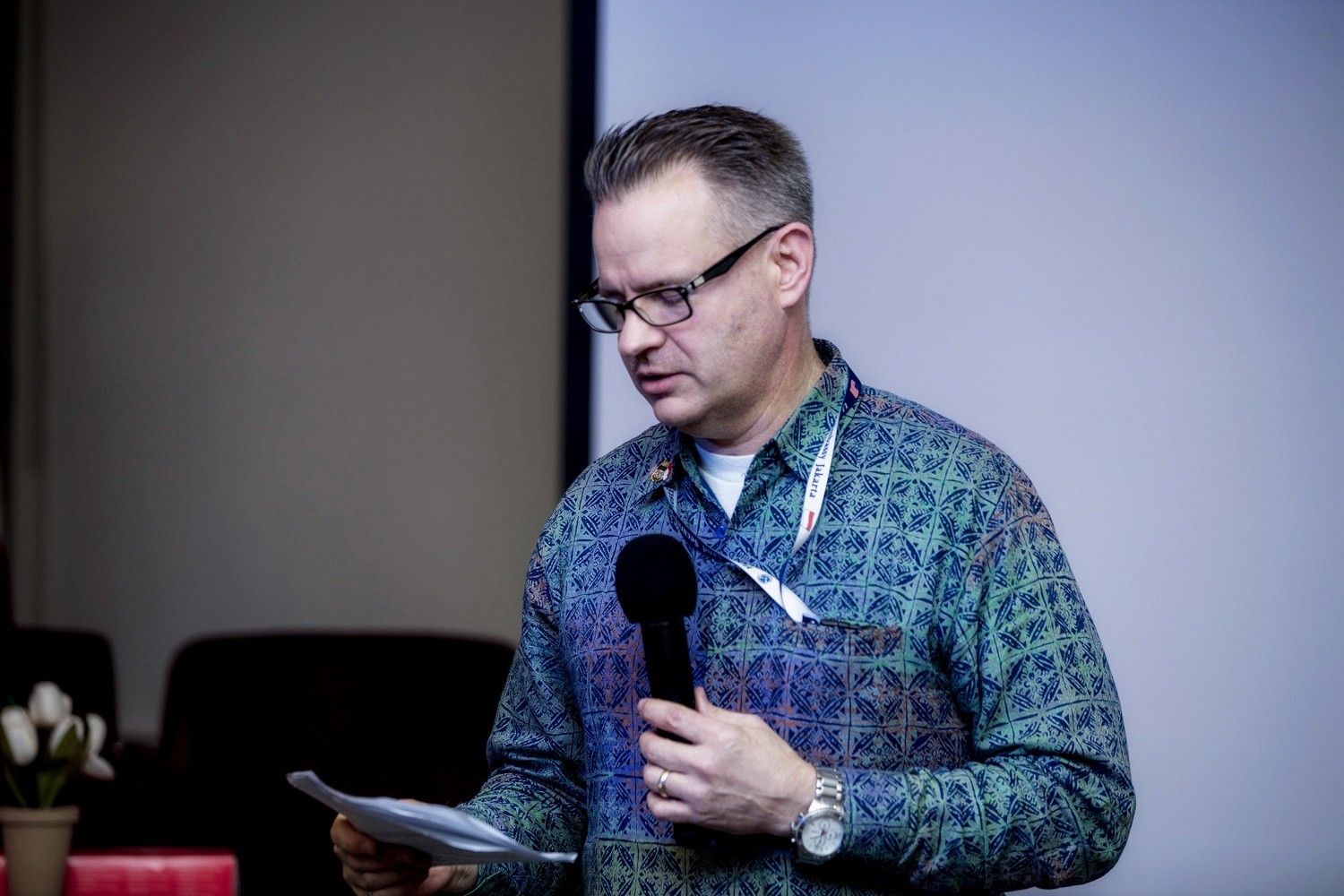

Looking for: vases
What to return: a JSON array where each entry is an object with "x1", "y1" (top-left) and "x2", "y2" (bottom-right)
[{"x1": 0, "y1": 803, "x2": 80, "y2": 896}]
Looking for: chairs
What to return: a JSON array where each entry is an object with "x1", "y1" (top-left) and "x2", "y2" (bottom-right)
[{"x1": 0, "y1": 621, "x2": 519, "y2": 896}]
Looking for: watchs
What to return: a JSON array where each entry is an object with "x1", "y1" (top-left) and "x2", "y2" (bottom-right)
[{"x1": 790, "y1": 765, "x2": 846, "y2": 865}]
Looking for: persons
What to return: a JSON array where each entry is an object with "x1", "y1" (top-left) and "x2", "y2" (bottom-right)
[{"x1": 330, "y1": 102, "x2": 1137, "y2": 894}]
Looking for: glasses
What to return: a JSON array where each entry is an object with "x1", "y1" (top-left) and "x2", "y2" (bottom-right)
[{"x1": 574, "y1": 223, "x2": 784, "y2": 333}]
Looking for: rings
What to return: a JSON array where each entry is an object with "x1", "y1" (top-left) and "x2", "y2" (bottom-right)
[{"x1": 657, "y1": 771, "x2": 670, "y2": 798}]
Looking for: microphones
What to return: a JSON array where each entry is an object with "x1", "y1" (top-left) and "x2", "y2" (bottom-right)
[{"x1": 614, "y1": 534, "x2": 713, "y2": 848}]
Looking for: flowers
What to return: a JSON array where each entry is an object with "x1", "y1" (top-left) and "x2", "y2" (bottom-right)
[{"x1": 0, "y1": 678, "x2": 115, "y2": 807}]
